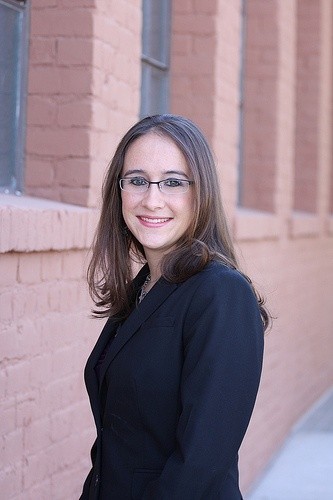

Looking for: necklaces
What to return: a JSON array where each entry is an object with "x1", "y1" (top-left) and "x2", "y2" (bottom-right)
[{"x1": 138, "y1": 274, "x2": 151, "y2": 300}]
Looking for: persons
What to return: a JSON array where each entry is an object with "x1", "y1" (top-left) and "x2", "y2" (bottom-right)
[{"x1": 78, "y1": 115, "x2": 269, "y2": 500}]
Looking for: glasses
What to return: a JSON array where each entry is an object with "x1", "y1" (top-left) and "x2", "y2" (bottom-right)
[{"x1": 119, "y1": 177, "x2": 194, "y2": 194}]
